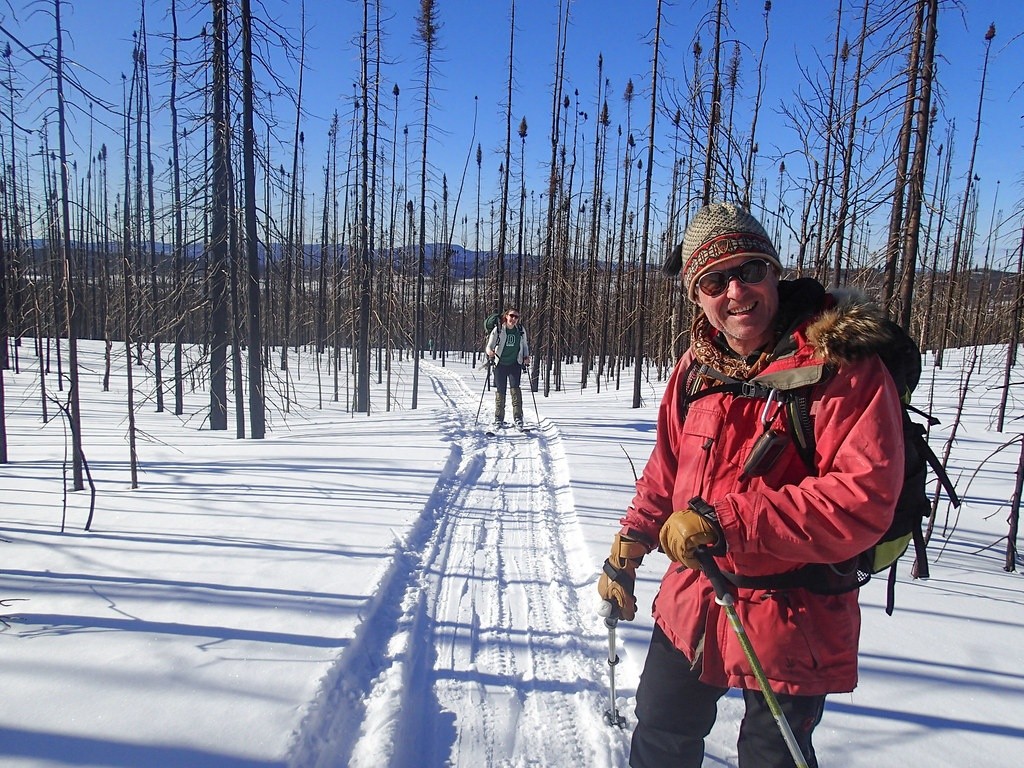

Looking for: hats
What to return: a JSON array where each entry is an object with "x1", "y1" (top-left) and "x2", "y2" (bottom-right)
[{"x1": 661, "y1": 202, "x2": 783, "y2": 303}]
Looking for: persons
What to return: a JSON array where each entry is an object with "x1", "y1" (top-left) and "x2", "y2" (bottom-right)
[
  {"x1": 598, "y1": 202, "x2": 922, "y2": 768},
  {"x1": 486, "y1": 308, "x2": 530, "y2": 432}
]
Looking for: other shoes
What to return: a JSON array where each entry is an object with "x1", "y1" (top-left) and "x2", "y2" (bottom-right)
[
  {"x1": 515, "y1": 418, "x2": 523, "y2": 427},
  {"x1": 492, "y1": 417, "x2": 501, "y2": 426}
]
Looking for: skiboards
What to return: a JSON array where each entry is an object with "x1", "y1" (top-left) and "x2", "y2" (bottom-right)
[{"x1": 485, "y1": 425, "x2": 531, "y2": 436}]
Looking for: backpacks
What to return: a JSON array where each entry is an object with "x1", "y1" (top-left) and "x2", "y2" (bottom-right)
[
  {"x1": 484, "y1": 313, "x2": 524, "y2": 345},
  {"x1": 681, "y1": 358, "x2": 932, "y2": 573}
]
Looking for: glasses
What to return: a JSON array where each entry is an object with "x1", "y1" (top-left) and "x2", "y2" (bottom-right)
[
  {"x1": 507, "y1": 314, "x2": 519, "y2": 319},
  {"x1": 695, "y1": 258, "x2": 771, "y2": 298}
]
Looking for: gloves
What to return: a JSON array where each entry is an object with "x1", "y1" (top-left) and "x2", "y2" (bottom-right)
[
  {"x1": 659, "y1": 510, "x2": 717, "y2": 569},
  {"x1": 598, "y1": 534, "x2": 646, "y2": 621}
]
